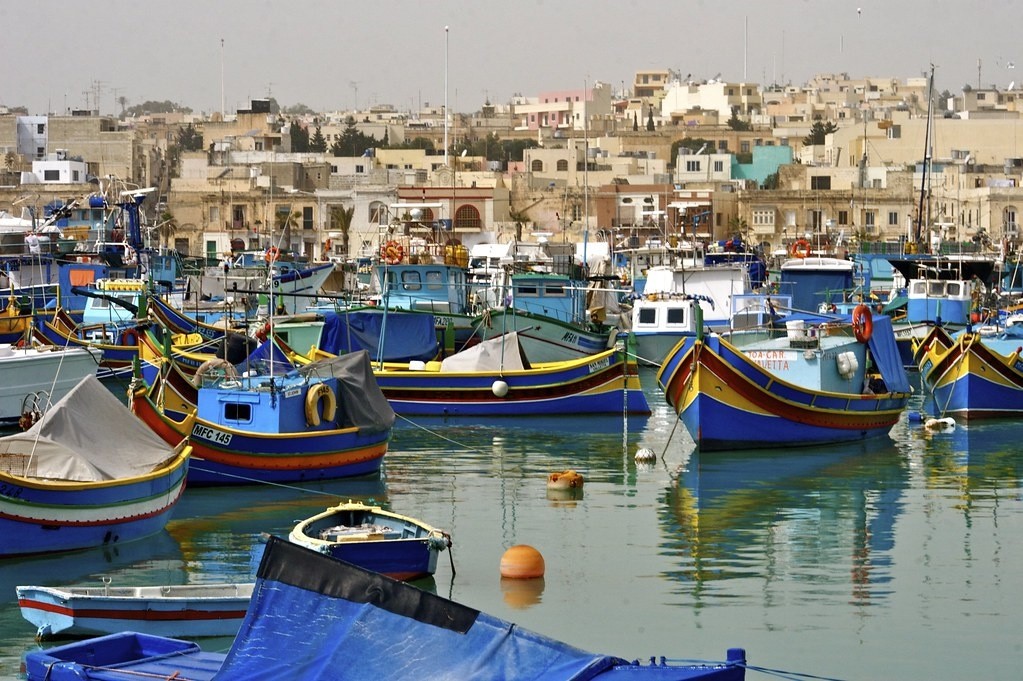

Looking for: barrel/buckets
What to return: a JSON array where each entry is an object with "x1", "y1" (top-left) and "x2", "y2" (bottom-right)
[{"x1": 785, "y1": 319, "x2": 804, "y2": 341}]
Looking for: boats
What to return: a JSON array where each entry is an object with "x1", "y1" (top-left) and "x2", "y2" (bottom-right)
[
  {"x1": 0, "y1": 354, "x2": 199, "y2": 559},
  {"x1": 16, "y1": 535, "x2": 747, "y2": 681},
  {"x1": 925, "y1": 313, "x2": 1023, "y2": 423},
  {"x1": 17, "y1": 493, "x2": 450, "y2": 642},
  {"x1": 285, "y1": 263, "x2": 654, "y2": 422},
  {"x1": 0, "y1": 63, "x2": 1022, "y2": 387},
  {"x1": 658, "y1": 289, "x2": 914, "y2": 451},
  {"x1": 129, "y1": 317, "x2": 395, "y2": 486}
]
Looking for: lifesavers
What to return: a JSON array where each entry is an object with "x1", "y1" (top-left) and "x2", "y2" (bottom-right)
[
  {"x1": 792, "y1": 240, "x2": 810, "y2": 259},
  {"x1": 852, "y1": 305, "x2": 873, "y2": 343},
  {"x1": 193, "y1": 358, "x2": 240, "y2": 388},
  {"x1": 977, "y1": 326, "x2": 1003, "y2": 335},
  {"x1": 305, "y1": 382, "x2": 337, "y2": 426},
  {"x1": 124, "y1": 246, "x2": 137, "y2": 266},
  {"x1": 267, "y1": 248, "x2": 280, "y2": 261},
  {"x1": 122, "y1": 329, "x2": 138, "y2": 347},
  {"x1": 380, "y1": 240, "x2": 404, "y2": 265}
]
